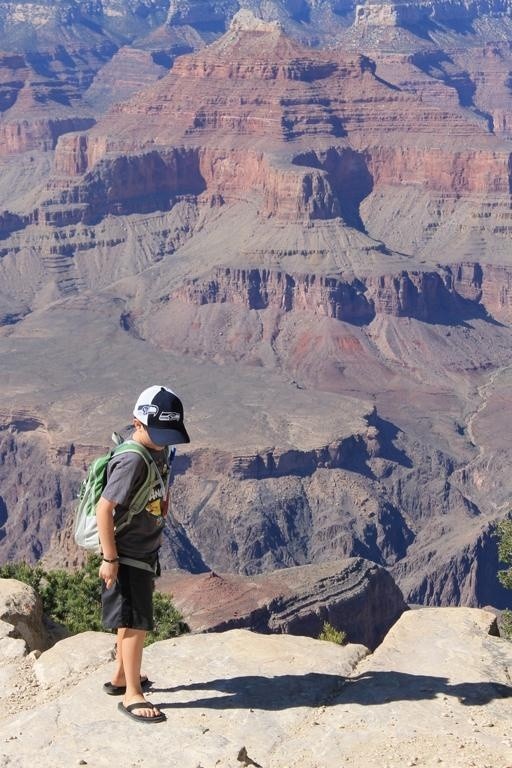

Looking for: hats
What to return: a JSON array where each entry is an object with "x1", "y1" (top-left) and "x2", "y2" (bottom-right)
[{"x1": 131, "y1": 383, "x2": 193, "y2": 447}]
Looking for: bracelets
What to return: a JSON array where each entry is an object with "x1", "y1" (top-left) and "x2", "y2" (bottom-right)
[{"x1": 103, "y1": 557, "x2": 120, "y2": 563}]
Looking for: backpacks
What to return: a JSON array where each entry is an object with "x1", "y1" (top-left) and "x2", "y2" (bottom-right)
[{"x1": 72, "y1": 431, "x2": 157, "y2": 556}]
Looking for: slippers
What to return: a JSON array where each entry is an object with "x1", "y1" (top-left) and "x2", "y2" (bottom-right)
[
  {"x1": 101, "y1": 667, "x2": 151, "y2": 695},
  {"x1": 116, "y1": 696, "x2": 168, "y2": 724}
]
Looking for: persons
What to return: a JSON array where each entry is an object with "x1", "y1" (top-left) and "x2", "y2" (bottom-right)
[{"x1": 94, "y1": 384, "x2": 193, "y2": 725}]
